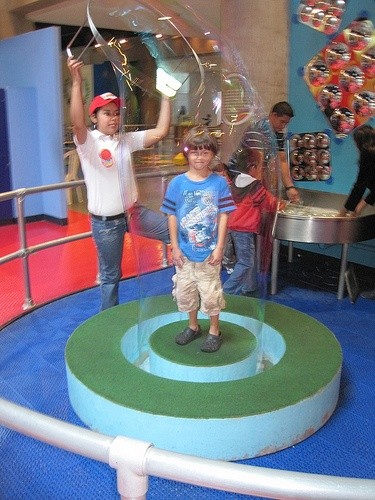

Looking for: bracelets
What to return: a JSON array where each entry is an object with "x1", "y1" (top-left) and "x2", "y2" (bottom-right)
[{"x1": 285, "y1": 186, "x2": 296, "y2": 192}]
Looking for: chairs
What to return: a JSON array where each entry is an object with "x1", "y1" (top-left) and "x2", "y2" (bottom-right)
[{"x1": 64, "y1": 149, "x2": 82, "y2": 205}]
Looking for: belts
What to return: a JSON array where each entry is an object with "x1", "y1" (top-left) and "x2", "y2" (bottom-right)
[{"x1": 91, "y1": 213, "x2": 124, "y2": 221}]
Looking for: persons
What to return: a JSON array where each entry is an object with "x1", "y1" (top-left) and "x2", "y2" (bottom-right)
[
  {"x1": 335, "y1": 124, "x2": 375, "y2": 217},
  {"x1": 221, "y1": 146, "x2": 285, "y2": 297},
  {"x1": 166, "y1": 124, "x2": 229, "y2": 353},
  {"x1": 221, "y1": 101, "x2": 301, "y2": 274},
  {"x1": 66, "y1": 55, "x2": 173, "y2": 310}
]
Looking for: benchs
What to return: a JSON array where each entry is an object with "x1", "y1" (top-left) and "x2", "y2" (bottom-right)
[{"x1": 166, "y1": 136, "x2": 222, "y2": 151}]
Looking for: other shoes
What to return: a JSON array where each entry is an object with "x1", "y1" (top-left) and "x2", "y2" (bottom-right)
[
  {"x1": 221, "y1": 261, "x2": 234, "y2": 274},
  {"x1": 360, "y1": 291, "x2": 375, "y2": 299},
  {"x1": 242, "y1": 290, "x2": 255, "y2": 297}
]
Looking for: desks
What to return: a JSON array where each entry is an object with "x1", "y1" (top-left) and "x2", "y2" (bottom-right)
[{"x1": 123, "y1": 123, "x2": 157, "y2": 132}]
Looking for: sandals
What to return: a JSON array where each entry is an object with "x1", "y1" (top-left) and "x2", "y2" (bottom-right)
[
  {"x1": 176, "y1": 324, "x2": 201, "y2": 345},
  {"x1": 201, "y1": 330, "x2": 222, "y2": 352}
]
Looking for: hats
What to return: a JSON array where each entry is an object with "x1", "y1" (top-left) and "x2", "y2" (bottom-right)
[{"x1": 89, "y1": 92, "x2": 126, "y2": 116}]
